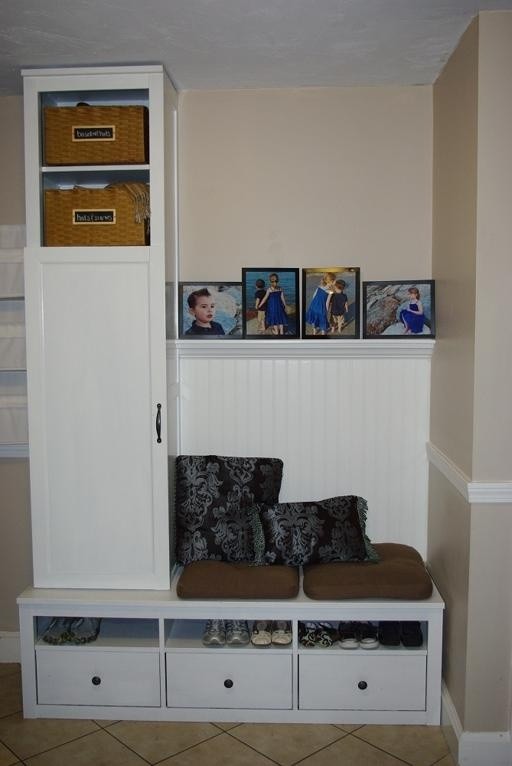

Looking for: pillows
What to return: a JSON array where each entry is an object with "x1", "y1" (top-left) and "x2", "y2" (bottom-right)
[
  {"x1": 303, "y1": 542, "x2": 432, "y2": 600},
  {"x1": 247, "y1": 494, "x2": 377, "y2": 566},
  {"x1": 177, "y1": 562, "x2": 300, "y2": 597},
  {"x1": 176, "y1": 455, "x2": 281, "y2": 566}
]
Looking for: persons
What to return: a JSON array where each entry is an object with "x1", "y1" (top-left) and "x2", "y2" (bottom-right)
[
  {"x1": 329, "y1": 279, "x2": 349, "y2": 332},
  {"x1": 399, "y1": 286, "x2": 425, "y2": 334},
  {"x1": 308, "y1": 272, "x2": 336, "y2": 335},
  {"x1": 252, "y1": 278, "x2": 269, "y2": 331},
  {"x1": 184, "y1": 288, "x2": 226, "y2": 336},
  {"x1": 258, "y1": 274, "x2": 289, "y2": 334}
]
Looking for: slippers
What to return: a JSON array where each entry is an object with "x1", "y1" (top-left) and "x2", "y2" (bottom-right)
[
  {"x1": 67, "y1": 619, "x2": 101, "y2": 642},
  {"x1": 42, "y1": 616, "x2": 71, "y2": 644}
]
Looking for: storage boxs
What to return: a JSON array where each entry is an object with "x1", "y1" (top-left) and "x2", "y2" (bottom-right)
[
  {"x1": 45, "y1": 189, "x2": 146, "y2": 245},
  {"x1": 42, "y1": 106, "x2": 147, "y2": 166}
]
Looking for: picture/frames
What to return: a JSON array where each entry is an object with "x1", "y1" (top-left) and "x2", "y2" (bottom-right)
[
  {"x1": 178, "y1": 282, "x2": 242, "y2": 339},
  {"x1": 301, "y1": 266, "x2": 359, "y2": 339},
  {"x1": 363, "y1": 280, "x2": 435, "y2": 338},
  {"x1": 242, "y1": 267, "x2": 300, "y2": 339}
]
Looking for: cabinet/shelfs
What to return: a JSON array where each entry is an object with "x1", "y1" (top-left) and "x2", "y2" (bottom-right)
[
  {"x1": 22, "y1": 65, "x2": 177, "y2": 591},
  {"x1": 16, "y1": 585, "x2": 444, "y2": 726}
]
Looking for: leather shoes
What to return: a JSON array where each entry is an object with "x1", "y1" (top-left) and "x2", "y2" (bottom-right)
[
  {"x1": 380, "y1": 621, "x2": 401, "y2": 645},
  {"x1": 402, "y1": 620, "x2": 422, "y2": 646}
]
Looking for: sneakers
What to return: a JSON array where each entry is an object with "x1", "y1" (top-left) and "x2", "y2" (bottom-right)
[
  {"x1": 273, "y1": 620, "x2": 292, "y2": 645},
  {"x1": 226, "y1": 620, "x2": 249, "y2": 644},
  {"x1": 202, "y1": 619, "x2": 226, "y2": 645},
  {"x1": 317, "y1": 622, "x2": 334, "y2": 647},
  {"x1": 302, "y1": 622, "x2": 317, "y2": 647},
  {"x1": 252, "y1": 620, "x2": 272, "y2": 646},
  {"x1": 339, "y1": 621, "x2": 359, "y2": 648},
  {"x1": 360, "y1": 622, "x2": 380, "y2": 649}
]
